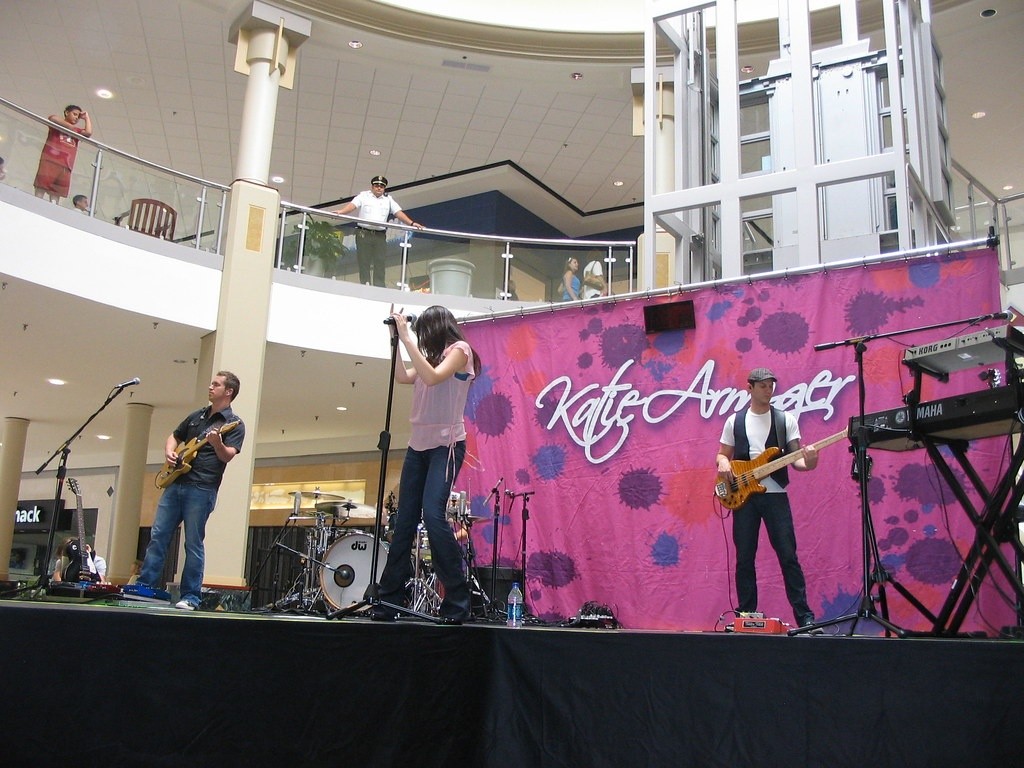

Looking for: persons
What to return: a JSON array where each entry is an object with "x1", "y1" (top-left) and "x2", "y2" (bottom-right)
[
  {"x1": 370, "y1": 302, "x2": 482, "y2": 625},
  {"x1": 558, "y1": 256, "x2": 580, "y2": 301},
  {"x1": 583, "y1": 250, "x2": 607, "y2": 300},
  {"x1": 384, "y1": 482, "x2": 467, "y2": 544},
  {"x1": 73, "y1": 195, "x2": 88, "y2": 214},
  {"x1": 54, "y1": 537, "x2": 106, "y2": 581},
  {"x1": 502, "y1": 279, "x2": 518, "y2": 301},
  {"x1": 136, "y1": 371, "x2": 245, "y2": 609},
  {"x1": 332, "y1": 175, "x2": 424, "y2": 288},
  {"x1": 716, "y1": 368, "x2": 823, "y2": 635},
  {"x1": 127, "y1": 560, "x2": 142, "y2": 585},
  {"x1": 33, "y1": 105, "x2": 92, "y2": 205}
]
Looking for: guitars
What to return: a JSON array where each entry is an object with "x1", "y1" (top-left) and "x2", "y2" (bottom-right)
[
  {"x1": 714, "y1": 425, "x2": 850, "y2": 510},
  {"x1": 61, "y1": 477, "x2": 102, "y2": 582},
  {"x1": 156, "y1": 419, "x2": 242, "y2": 487}
]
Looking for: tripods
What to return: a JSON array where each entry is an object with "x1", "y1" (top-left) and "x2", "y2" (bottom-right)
[
  {"x1": 273, "y1": 524, "x2": 331, "y2": 617},
  {"x1": 326, "y1": 323, "x2": 447, "y2": 625},
  {"x1": 0, "y1": 387, "x2": 125, "y2": 598},
  {"x1": 786, "y1": 315, "x2": 993, "y2": 639},
  {"x1": 466, "y1": 490, "x2": 548, "y2": 628}
]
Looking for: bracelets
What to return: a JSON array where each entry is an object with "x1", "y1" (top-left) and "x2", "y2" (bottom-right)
[{"x1": 411, "y1": 221, "x2": 415, "y2": 226}]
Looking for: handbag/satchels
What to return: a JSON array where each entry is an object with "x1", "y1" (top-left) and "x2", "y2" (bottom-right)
[
  {"x1": 583, "y1": 271, "x2": 605, "y2": 291},
  {"x1": 558, "y1": 283, "x2": 564, "y2": 296}
]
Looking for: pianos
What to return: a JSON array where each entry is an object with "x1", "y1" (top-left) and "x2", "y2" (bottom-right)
[{"x1": 847, "y1": 380, "x2": 1024, "y2": 640}]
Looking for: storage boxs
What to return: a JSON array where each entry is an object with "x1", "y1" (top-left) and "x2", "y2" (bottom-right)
[{"x1": 429, "y1": 259, "x2": 475, "y2": 297}]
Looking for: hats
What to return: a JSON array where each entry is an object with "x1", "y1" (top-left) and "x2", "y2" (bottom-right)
[
  {"x1": 749, "y1": 368, "x2": 777, "y2": 382},
  {"x1": 372, "y1": 176, "x2": 389, "y2": 187}
]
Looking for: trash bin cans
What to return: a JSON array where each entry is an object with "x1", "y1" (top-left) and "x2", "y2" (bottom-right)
[{"x1": 471, "y1": 564, "x2": 522, "y2": 607}]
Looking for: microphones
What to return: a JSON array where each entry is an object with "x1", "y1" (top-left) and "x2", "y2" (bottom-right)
[
  {"x1": 340, "y1": 569, "x2": 350, "y2": 580},
  {"x1": 115, "y1": 377, "x2": 141, "y2": 389},
  {"x1": 483, "y1": 477, "x2": 504, "y2": 507},
  {"x1": 384, "y1": 314, "x2": 418, "y2": 325},
  {"x1": 986, "y1": 310, "x2": 1014, "y2": 320}
]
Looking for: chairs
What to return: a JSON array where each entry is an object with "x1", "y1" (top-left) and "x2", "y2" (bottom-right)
[{"x1": 116, "y1": 198, "x2": 178, "y2": 242}]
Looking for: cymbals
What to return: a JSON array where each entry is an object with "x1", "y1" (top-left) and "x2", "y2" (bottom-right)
[
  {"x1": 288, "y1": 490, "x2": 346, "y2": 500},
  {"x1": 456, "y1": 515, "x2": 490, "y2": 523},
  {"x1": 315, "y1": 502, "x2": 384, "y2": 519}
]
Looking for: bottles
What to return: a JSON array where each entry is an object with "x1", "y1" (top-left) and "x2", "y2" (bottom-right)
[{"x1": 507, "y1": 583, "x2": 522, "y2": 627}]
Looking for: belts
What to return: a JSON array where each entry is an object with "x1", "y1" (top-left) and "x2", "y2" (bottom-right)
[{"x1": 357, "y1": 225, "x2": 386, "y2": 234}]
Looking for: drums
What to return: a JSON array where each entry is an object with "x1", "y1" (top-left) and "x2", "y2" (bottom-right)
[
  {"x1": 411, "y1": 528, "x2": 432, "y2": 555},
  {"x1": 318, "y1": 531, "x2": 416, "y2": 616}
]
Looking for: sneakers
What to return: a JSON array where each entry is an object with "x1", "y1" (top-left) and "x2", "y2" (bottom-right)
[
  {"x1": 727, "y1": 622, "x2": 755, "y2": 632},
  {"x1": 176, "y1": 598, "x2": 196, "y2": 610},
  {"x1": 805, "y1": 621, "x2": 824, "y2": 634}
]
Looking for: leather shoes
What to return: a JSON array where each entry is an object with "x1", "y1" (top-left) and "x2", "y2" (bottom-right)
[
  {"x1": 438, "y1": 614, "x2": 462, "y2": 625},
  {"x1": 370, "y1": 613, "x2": 391, "y2": 621}
]
